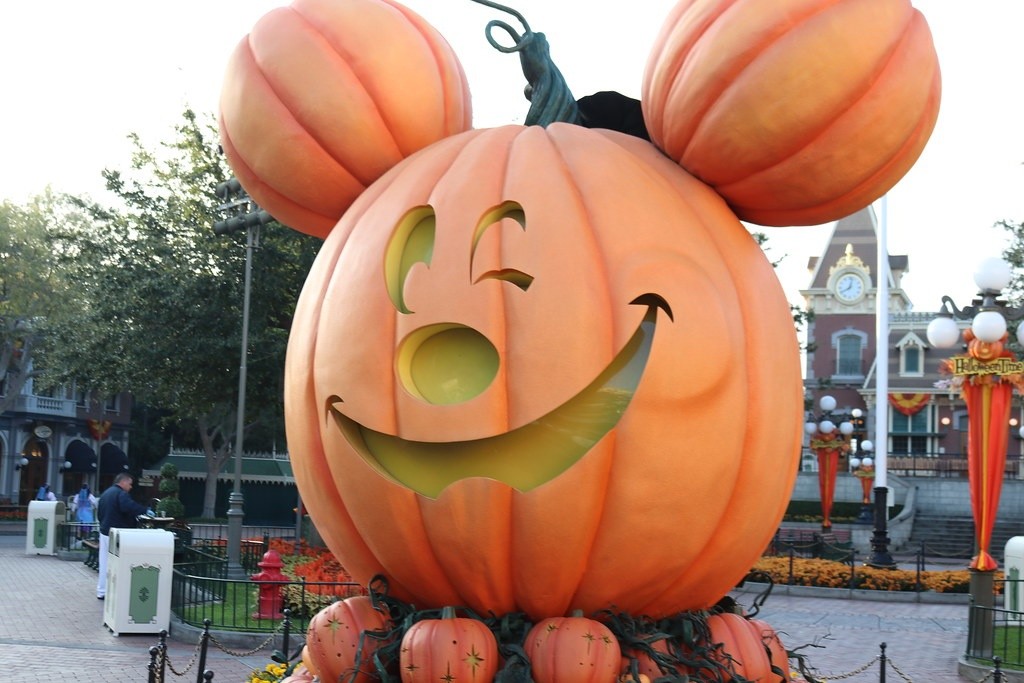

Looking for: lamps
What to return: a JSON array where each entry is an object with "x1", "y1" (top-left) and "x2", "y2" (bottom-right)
[
  {"x1": 59, "y1": 461, "x2": 72, "y2": 474},
  {"x1": 15, "y1": 458, "x2": 28, "y2": 470},
  {"x1": 88, "y1": 463, "x2": 97, "y2": 472}
]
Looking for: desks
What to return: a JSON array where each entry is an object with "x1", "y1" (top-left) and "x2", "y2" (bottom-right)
[{"x1": 60, "y1": 521, "x2": 100, "y2": 551}]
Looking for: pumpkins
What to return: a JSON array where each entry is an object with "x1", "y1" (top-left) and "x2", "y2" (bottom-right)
[
  {"x1": 641, "y1": 0, "x2": 942, "y2": 228},
  {"x1": 219, "y1": 0, "x2": 474, "y2": 240}
]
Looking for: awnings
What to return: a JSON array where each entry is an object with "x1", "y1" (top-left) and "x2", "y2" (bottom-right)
[{"x1": 141, "y1": 450, "x2": 297, "y2": 486}]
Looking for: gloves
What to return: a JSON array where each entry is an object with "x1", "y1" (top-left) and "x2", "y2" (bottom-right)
[{"x1": 146, "y1": 510, "x2": 155, "y2": 518}]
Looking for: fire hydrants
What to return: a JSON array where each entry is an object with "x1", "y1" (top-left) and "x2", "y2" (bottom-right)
[{"x1": 250, "y1": 550, "x2": 290, "y2": 619}]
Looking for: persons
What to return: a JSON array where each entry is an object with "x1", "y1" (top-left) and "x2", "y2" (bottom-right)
[
  {"x1": 71, "y1": 483, "x2": 98, "y2": 540},
  {"x1": 35, "y1": 482, "x2": 57, "y2": 501},
  {"x1": 97, "y1": 473, "x2": 146, "y2": 599}
]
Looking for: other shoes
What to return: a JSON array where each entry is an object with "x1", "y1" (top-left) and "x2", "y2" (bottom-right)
[{"x1": 97, "y1": 595, "x2": 104, "y2": 600}]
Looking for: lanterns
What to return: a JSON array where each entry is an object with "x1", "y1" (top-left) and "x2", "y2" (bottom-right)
[{"x1": 219, "y1": 1, "x2": 941, "y2": 619}]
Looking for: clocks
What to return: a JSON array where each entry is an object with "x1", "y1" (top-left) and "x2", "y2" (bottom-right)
[
  {"x1": 33, "y1": 426, "x2": 53, "y2": 438},
  {"x1": 832, "y1": 268, "x2": 868, "y2": 305}
]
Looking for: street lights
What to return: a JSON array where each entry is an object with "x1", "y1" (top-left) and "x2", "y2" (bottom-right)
[
  {"x1": 927, "y1": 259, "x2": 1024, "y2": 660},
  {"x1": 850, "y1": 440, "x2": 875, "y2": 503},
  {"x1": 805, "y1": 396, "x2": 853, "y2": 533}
]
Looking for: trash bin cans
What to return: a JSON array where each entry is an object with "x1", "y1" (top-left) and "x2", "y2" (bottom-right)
[
  {"x1": 1003, "y1": 535, "x2": 1024, "y2": 625},
  {"x1": 103, "y1": 526, "x2": 175, "y2": 637},
  {"x1": 25, "y1": 499, "x2": 66, "y2": 556}
]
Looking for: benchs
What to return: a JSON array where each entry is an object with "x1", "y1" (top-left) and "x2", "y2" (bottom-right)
[{"x1": 82, "y1": 529, "x2": 99, "y2": 573}]
